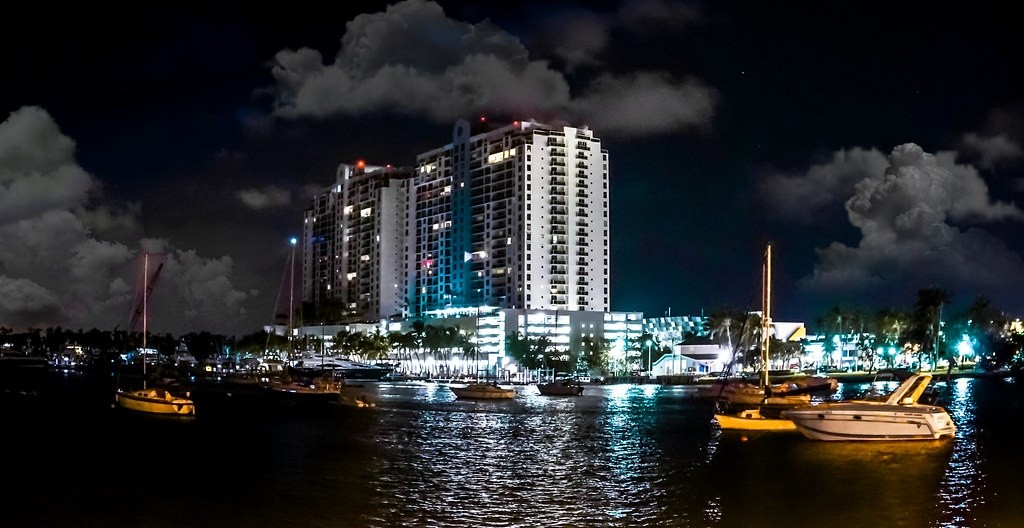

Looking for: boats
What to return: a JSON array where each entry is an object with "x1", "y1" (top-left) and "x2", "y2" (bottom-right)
[
  {"x1": 290, "y1": 353, "x2": 393, "y2": 383},
  {"x1": 780, "y1": 398, "x2": 957, "y2": 441},
  {"x1": 697, "y1": 374, "x2": 839, "y2": 401}
]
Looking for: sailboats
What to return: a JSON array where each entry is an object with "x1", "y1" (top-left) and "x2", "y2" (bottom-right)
[
  {"x1": 114, "y1": 254, "x2": 195, "y2": 415},
  {"x1": 710, "y1": 244, "x2": 933, "y2": 431},
  {"x1": 257, "y1": 240, "x2": 340, "y2": 399},
  {"x1": 536, "y1": 310, "x2": 585, "y2": 395},
  {"x1": 448, "y1": 306, "x2": 518, "y2": 400}
]
